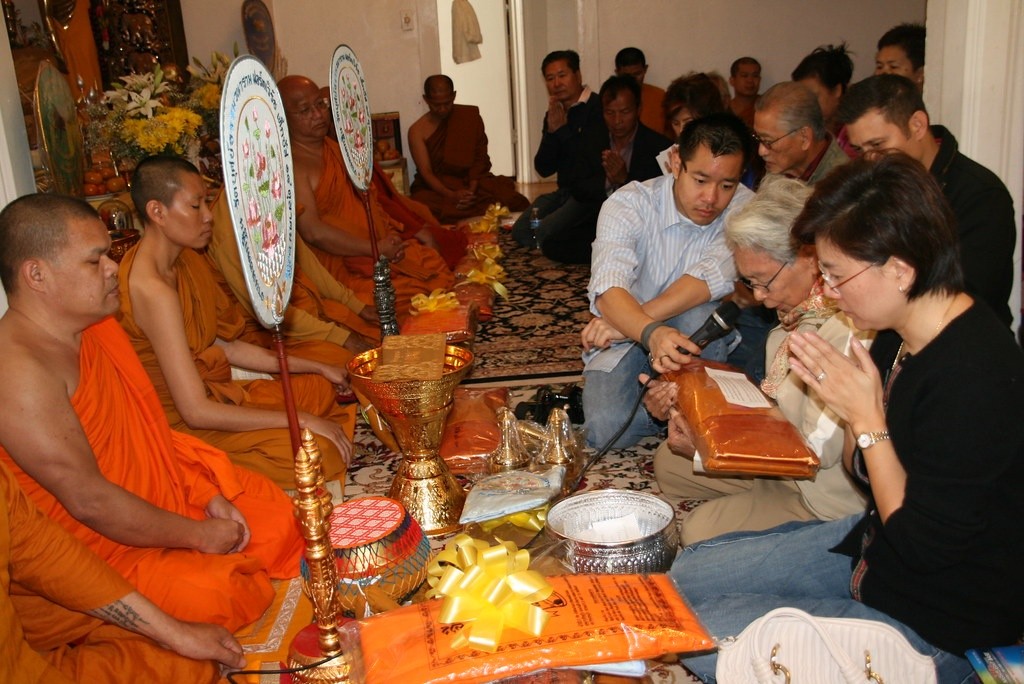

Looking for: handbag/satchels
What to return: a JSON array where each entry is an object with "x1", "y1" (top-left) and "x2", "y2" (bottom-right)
[{"x1": 717, "y1": 608, "x2": 936, "y2": 683}]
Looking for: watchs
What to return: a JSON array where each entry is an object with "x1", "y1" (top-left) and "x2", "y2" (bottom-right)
[{"x1": 856, "y1": 431, "x2": 891, "y2": 450}]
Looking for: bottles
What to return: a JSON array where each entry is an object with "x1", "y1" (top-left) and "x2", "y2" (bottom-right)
[{"x1": 530, "y1": 208, "x2": 543, "y2": 250}]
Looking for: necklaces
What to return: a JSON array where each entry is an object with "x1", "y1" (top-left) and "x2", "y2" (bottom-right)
[{"x1": 892, "y1": 291, "x2": 961, "y2": 371}]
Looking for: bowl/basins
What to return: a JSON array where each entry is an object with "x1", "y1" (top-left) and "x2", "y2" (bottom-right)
[{"x1": 545, "y1": 488, "x2": 678, "y2": 575}]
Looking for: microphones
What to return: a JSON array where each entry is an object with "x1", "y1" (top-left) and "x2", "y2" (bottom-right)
[{"x1": 651, "y1": 302, "x2": 741, "y2": 380}]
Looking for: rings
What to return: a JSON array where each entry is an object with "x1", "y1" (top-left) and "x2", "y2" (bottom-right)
[
  {"x1": 648, "y1": 355, "x2": 656, "y2": 366},
  {"x1": 816, "y1": 372, "x2": 827, "y2": 382},
  {"x1": 660, "y1": 355, "x2": 670, "y2": 366}
]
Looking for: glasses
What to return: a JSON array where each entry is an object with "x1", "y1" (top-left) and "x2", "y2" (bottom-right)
[
  {"x1": 753, "y1": 127, "x2": 799, "y2": 149},
  {"x1": 737, "y1": 261, "x2": 789, "y2": 293},
  {"x1": 817, "y1": 259, "x2": 881, "y2": 292},
  {"x1": 286, "y1": 97, "x2": 330, "y2": 119}
]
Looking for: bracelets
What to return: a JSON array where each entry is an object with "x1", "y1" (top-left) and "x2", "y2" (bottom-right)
[{"x1": 641, "y1": 321, "x2": 676, "y2": 352}]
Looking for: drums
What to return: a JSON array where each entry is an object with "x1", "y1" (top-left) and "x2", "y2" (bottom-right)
[{"x1": 299, "y1": 496, "x2": 435, "y2": 618}]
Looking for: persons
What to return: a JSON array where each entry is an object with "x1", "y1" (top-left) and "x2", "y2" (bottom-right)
[
  {"x1": 200, "y1": 74, "x2": 456, "y2": 358},
  {"x1": 0, "y1": 460, "x2": 251, "y2": 684},
  {"x1": 0, "y1": 191, "x2": 309, "y2": 639},
  {"x1": 407, "y1": 74, "x2": 532, "y2": 224},
  {"x1": 580, "y1": 117, "x2": 758, "y2": 452},
  {"x1": 665, "y1": 148, "x2": 1024, "y2": 684},
  {"x1": 511, "y1": 23, "x2": 1017, "y2": 389},
  {"x1": 638, "y1": 172, "x2": 885, "y2": 548},
  {"x1": 116, "y1": 154, "x2": 353, "y2": 492}
]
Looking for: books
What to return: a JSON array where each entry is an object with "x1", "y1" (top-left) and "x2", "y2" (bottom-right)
[{"x1": 965, "y1": 646, "x2": 1024, "y2": 684}]
